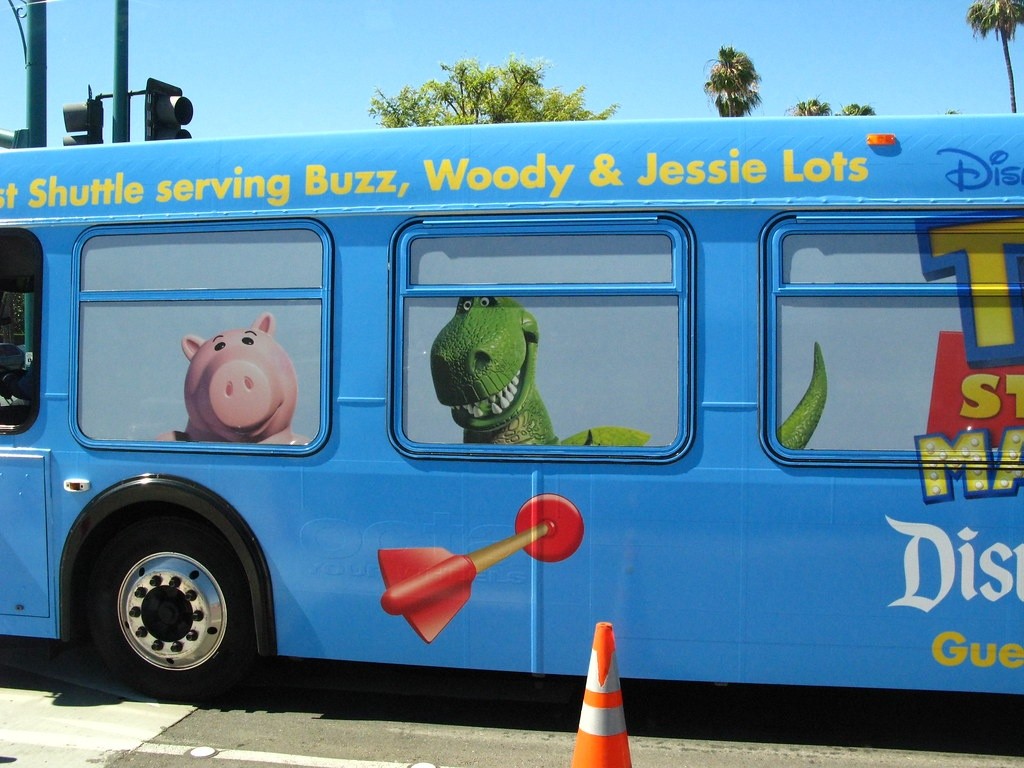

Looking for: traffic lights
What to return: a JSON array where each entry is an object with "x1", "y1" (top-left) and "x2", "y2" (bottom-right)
[
  {"x1": 0, "y1": 127, "x2": 28, "y2": 149},
  {"x1": 144, "y1": 78, "x2": 194, "y2": 141},
  {"x1": 62, "y1": 84, "x2": 104, "y2": 146}
]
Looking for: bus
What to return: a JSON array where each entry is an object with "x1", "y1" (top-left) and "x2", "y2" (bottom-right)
[{"x1": 0, "y1": 116, "x2": 1024, "y2": 695}]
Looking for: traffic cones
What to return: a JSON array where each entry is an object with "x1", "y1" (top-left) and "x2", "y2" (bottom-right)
[{"x1": 569, "y1": 621, "x2": 636, "y2": 768}]
